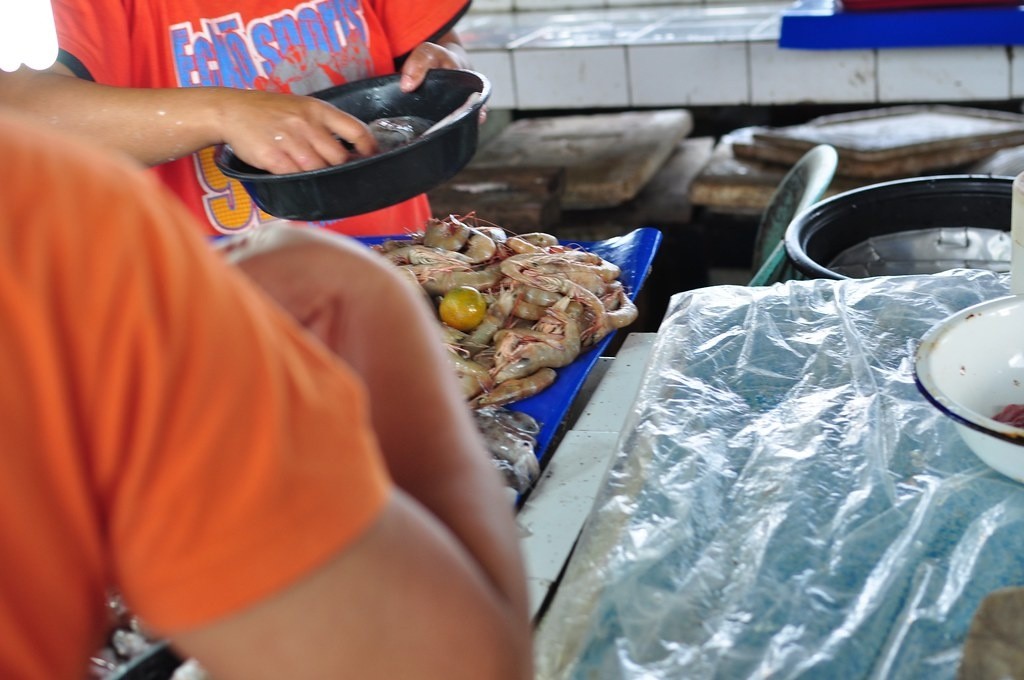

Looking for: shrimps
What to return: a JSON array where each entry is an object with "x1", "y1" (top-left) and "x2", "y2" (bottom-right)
[{"x1": 386, "y1": 211, "x2": 638, "y2": 496}]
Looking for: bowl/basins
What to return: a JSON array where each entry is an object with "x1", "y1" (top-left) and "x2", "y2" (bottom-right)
[
  {"x1": 784, "y1": 171, "x2": 1015, "y2": 282},
  {"x1": 911, "y1": 294, "x2": 1023, "y2": 484},
  {"x1": 212, "y1": 68, "x2": 493, "y2": 221}
]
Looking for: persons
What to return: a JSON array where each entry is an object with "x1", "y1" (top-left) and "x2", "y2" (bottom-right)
[
  {"x1": 0, "y1": 113, "x2": 533, "y2": 680},
  {"x1": 0, "y1": 0, "x2": 470, "y2": 241}
]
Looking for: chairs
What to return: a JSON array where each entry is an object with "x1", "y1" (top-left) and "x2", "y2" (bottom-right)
[{"x1": 743, "y1": 145, "x2": 842, "y2": 287}]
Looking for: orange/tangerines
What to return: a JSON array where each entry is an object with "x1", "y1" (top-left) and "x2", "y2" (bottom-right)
[{"x1": 439, "y1": 286, "x2": 486, "y2": 331}]
